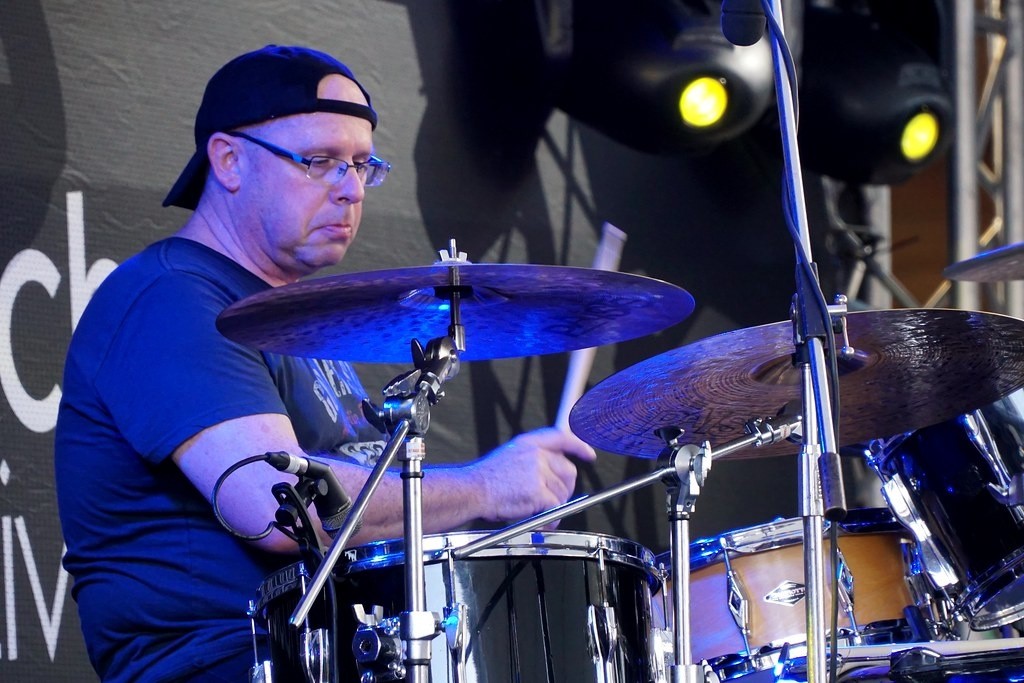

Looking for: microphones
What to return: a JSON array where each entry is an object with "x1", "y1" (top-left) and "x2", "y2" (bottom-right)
[
  {"x1": 721, "y1": 0, "x2": 766, "y2": 46},
  {"x1": 265, "y1": 450, "x2": 365, "y2": 540}
]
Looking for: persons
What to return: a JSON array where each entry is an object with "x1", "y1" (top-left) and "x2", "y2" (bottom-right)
[{"x1": 52, "y1": 44, "x2": 596, "y2": 683}]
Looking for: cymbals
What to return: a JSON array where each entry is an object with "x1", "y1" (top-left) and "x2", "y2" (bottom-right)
[
  {"x1": 214, "y1": 260, "x2": 700, "y2": 369},
  {"x1": 565, "y1": 306, "x2": 1024, "y2": 462},
  {"x1": 940, "y1": 240, "x2": 1024, "y2": 283}
]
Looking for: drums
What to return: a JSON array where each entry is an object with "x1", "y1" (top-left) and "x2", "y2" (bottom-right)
[
  {"x1": 648, "y1": 503, "x2": 942, "y2": 683},
  {"x1": 260, "y1": 525, "x2": 670, "y2": 683},
  {"x1": 866, "y1": 385, "x2": 1024, "y2": 635}
]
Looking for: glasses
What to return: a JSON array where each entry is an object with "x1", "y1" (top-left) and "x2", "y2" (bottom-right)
[{"x1": 224, "y1": 130, "x2": 390, "y2": 186}]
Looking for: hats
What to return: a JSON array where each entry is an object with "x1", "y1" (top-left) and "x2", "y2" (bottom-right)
[{"x1": 162, "y1": 44, "x2": 378, "y2": 211}]
